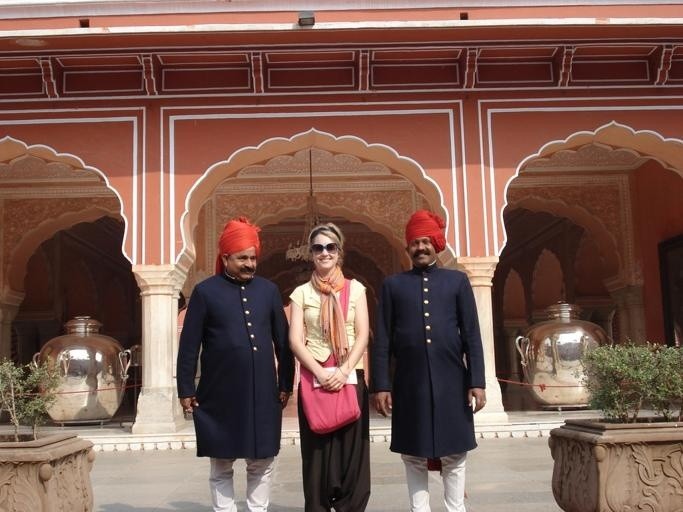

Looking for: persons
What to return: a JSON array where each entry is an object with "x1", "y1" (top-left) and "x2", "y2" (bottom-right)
[
  {"x1": 286, "y1": 222, "x2": 372, "y2": 512},
  {"x1": 176, "y1": 215, "x2": 298, "y2": 511},
  {"x1": 368, "y1": 208, "x2": 488, "y2": 512}
]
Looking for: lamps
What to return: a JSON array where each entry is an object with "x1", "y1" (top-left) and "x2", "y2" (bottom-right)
[{"x1": 297, "y1": 11, "x2": 315, "y2": 26}]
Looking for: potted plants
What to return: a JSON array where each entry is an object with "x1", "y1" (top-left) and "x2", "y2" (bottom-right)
[
  {"x1": 0, "y1": 357, "x2": 95, "y2": 512},
  {"x1": 549, "y1": 336, "x2": 683, "y2": 512}
]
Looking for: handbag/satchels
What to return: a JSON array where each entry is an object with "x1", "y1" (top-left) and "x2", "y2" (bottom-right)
[{"x1": 299, "y1": 349, "x2": 358, "y2": 435}]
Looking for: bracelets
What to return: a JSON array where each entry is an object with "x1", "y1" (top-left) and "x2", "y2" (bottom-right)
[{"x1": 340, "y1": 366, "x2": 349, "y2": 379}]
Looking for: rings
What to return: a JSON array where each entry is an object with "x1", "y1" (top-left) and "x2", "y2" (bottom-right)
[{"x1": 483, "y1": 400, "x2": 485, "y2": 404}]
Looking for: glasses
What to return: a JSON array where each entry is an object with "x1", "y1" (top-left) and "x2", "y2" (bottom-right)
[{"x1": 310, "y1": 241, "x2": 338, "y2": 256}]
